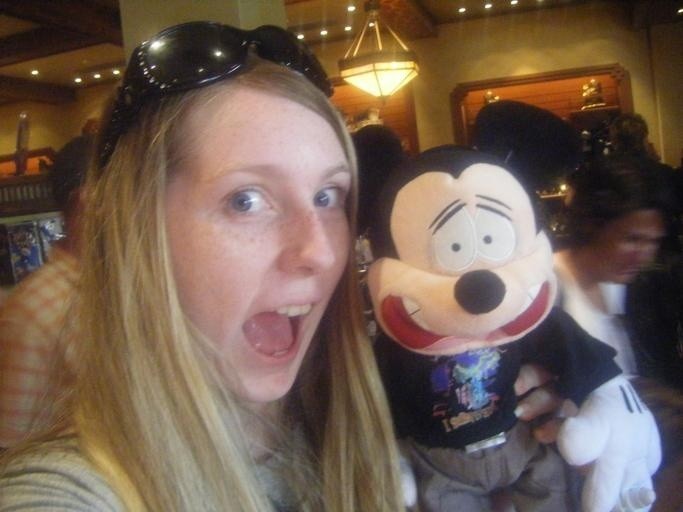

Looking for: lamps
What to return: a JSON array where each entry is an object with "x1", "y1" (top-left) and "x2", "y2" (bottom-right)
[{"x1": 338, "y1": 0, "x2": 420, "y2": 105}]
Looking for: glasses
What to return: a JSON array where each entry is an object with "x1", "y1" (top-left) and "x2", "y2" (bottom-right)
[{"x1": 97, "y1": 19, "x2": 335, "y2": 155}]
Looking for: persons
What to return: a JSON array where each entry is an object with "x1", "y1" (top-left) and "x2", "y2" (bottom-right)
[
  {"x1": 630, "y1": 377, "x2": 681, "y2": 511},
  {"x1": 0, "y1": 135, "x2": 88, "y2": 455},
  {"x1": 551, "y1": 153, "x2": 666, "y2": 499},
  {"x1": 1, "y1": 20, "x2": 566, "y2": 511}
]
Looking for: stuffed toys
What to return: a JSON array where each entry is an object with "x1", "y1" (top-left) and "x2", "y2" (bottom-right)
[{"x1": 348, "y1": 101, "x2": 664, "y2": 511}]
